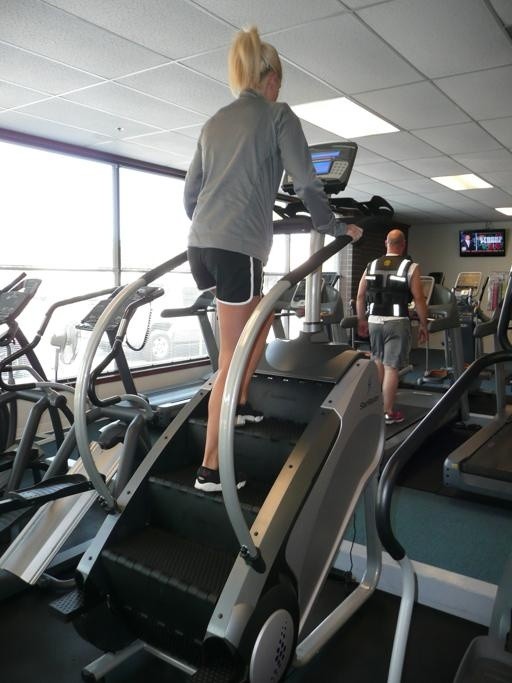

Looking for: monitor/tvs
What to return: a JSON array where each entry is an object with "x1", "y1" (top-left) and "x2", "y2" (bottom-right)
[{"x1": 459, "y1": 229, "x2": 506, "y2": 257}]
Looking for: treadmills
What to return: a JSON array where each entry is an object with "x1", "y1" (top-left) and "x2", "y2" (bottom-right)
[{"x1": 103, "y1": 272, "x2": 512, "y2": 504}]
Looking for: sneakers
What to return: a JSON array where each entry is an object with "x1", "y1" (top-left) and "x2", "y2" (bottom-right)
[
  {"x1": 194, "y1": 469, "x2": 243, "y2": 491},
  {"x1": 384, "y1": 411, "x2": 405, "y2": 424},
  {"x1": 234, "y1": 403, "x2": 263, "y2": 427}
]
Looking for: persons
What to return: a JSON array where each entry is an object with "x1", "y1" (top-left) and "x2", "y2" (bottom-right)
[
  {"x1": 184, "y1": 27, "x2": 364, "y2": 495},
  {"x1": 461, "y1": 233, "x2": 475, "y2": 251},
  {"x1": 356, "y1": 230, "x2": 429, "y2": 425}
]
